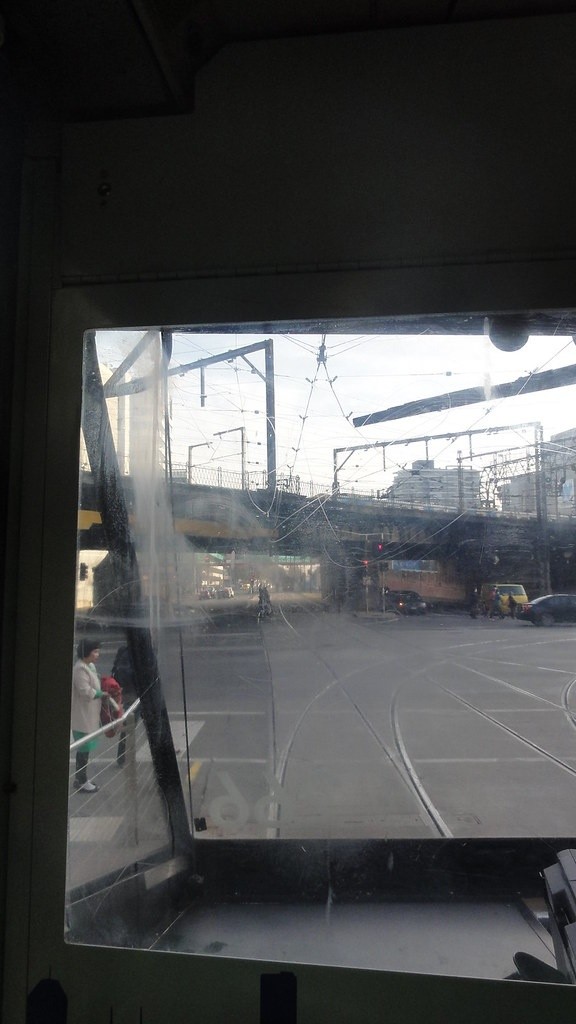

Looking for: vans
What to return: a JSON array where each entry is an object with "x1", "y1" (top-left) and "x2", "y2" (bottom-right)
[{"x1": 480, "y1": 584, "x2": 529, "y2": 615}]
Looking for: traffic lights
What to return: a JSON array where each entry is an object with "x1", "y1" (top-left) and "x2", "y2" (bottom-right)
[{"x1": 376, "y1": 541, "x2": 384, "y2": 558}]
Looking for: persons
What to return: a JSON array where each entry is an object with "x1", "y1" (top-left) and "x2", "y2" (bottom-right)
[
  {"x1": 508, "y1": 591, "x2": 517, "y2": 620},
  {"x1": 470, "y1": 587, "x2": 480, "y2": 619},
  {"x1": 71, "y1": 636, "x2": 113, "y2": 793},
  {"x1": 487, "y1": 587, "x2": 505, "y2": 622},
  {"x1": 112, "y1": 623, "x2": 181, "y2": 764},
  {"x1": 258, "y1": 587, "x2": 274, "y2": 621}
]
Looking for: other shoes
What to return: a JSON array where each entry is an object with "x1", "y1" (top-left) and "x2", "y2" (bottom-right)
[{"x1": 73, "y1": 780, "x2": 98, "y2": 793}]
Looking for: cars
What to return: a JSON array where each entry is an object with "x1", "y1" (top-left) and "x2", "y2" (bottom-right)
[
  {"x1": 384, "y1": 589, "x2": 427, "y2": 614},
  {"x1": 517, "y1": 593, "x2": 576, "y2": 627}
]
